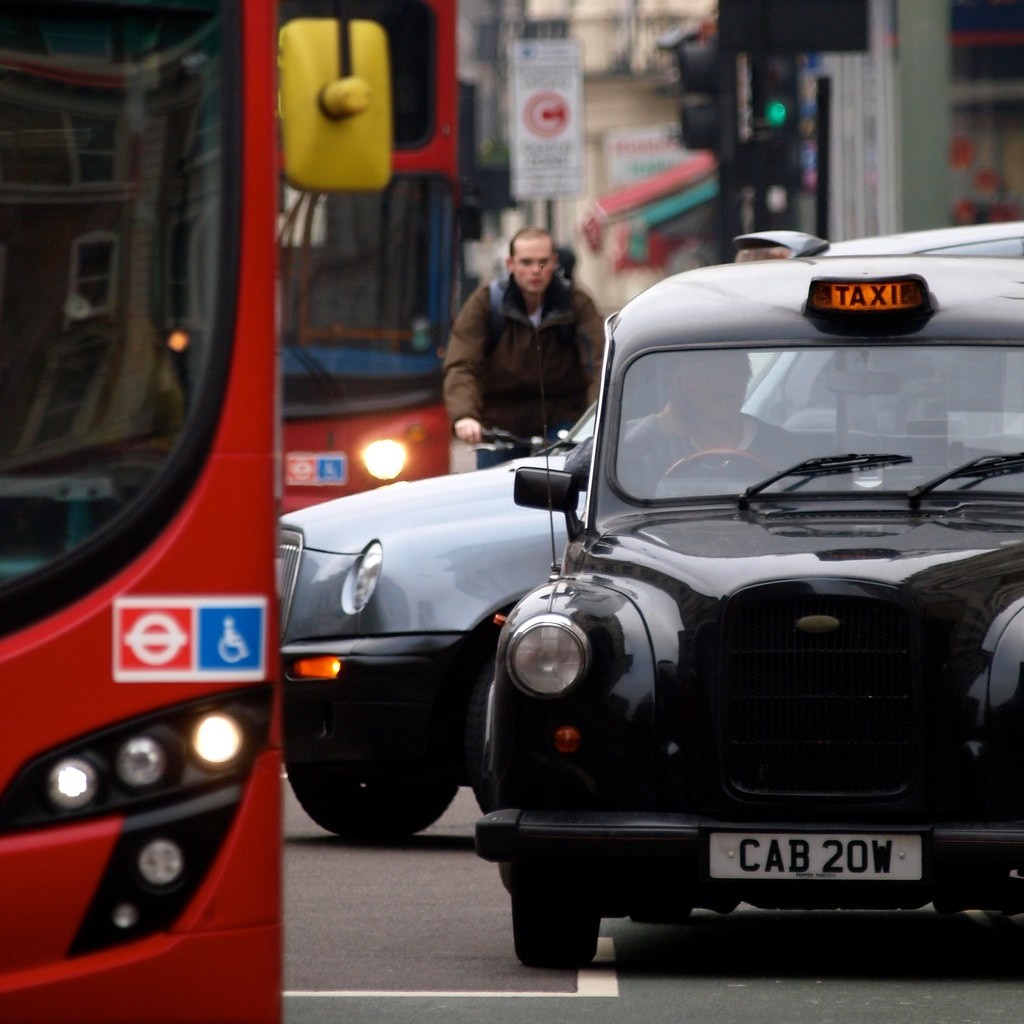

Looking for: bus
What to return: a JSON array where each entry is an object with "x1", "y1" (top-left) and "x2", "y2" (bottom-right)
[{"x1": 0, "y1": 0, "x2": 456, "y2": 1023}]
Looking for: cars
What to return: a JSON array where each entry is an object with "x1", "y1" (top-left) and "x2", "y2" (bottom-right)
[{"x1": 276, "y1": 220, "x2": 1024, "y2": 968}]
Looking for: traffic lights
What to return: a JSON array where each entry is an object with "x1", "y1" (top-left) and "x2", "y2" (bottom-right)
[
  {"x1": 676, "y1": 41, "x2": 750, "y2": 169},
  {"x1": 750, "y1": 86, "x2": 799, "y2": 230}
]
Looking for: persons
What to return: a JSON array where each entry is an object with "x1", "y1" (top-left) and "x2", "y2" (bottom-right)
[
  {"x1": 443, "y1": 227, "x2": 606, "y2": 471},
  {"x1": 562, "y1": 352, "x2": 805, "y2": 499}
]
